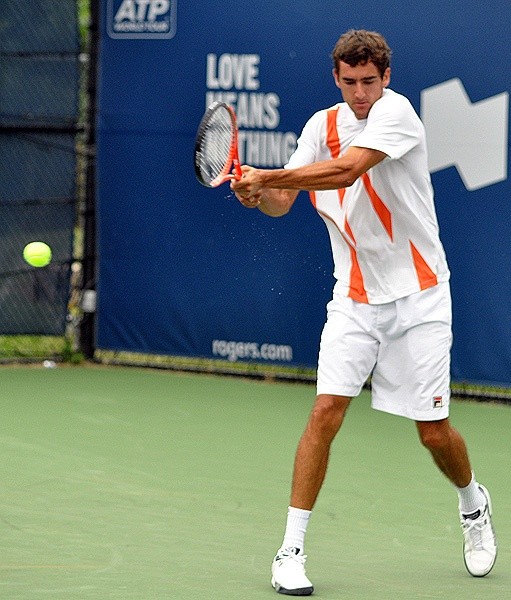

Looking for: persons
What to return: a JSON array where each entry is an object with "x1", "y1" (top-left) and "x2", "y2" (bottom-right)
[{"x1": 230, "y1": 27, "x2": 498, "y2": 598}]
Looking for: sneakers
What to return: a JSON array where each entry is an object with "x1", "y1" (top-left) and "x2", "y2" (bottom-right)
[
  {"x1": 270, "y1": 550, "x2": 314, "y2": 597},
  {"x1": 458, "y1": 484, "x2": 499, "y2": 577}
]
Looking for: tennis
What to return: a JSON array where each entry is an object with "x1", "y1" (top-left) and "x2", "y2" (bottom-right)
[{"x1": 25, "y1": 241, "x2": 53, "y2": 266}]
